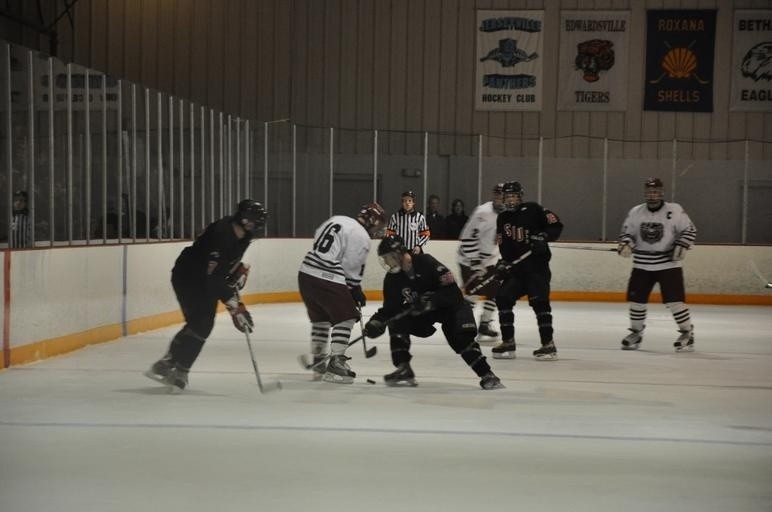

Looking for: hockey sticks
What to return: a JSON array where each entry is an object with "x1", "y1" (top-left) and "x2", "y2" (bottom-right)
[
  {"x1": 297, "y1": 307, "x2": 411, "y2": 372},
  {"x1": 233, "y1": 286, "x2": 285, "y2": 394},
  {"x1": 355, "y1": 299, "x2": 377, "y2": 359}
]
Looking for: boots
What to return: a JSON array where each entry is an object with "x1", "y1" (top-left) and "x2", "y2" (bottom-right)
[
  {"x1": 476, "y1": 321, "x2": 501, "y2": 344},
  {"x1": 384, "y1": 364, "x2": 418, "y2": 387},
  {"x1": 145, "y1": 354, "x2": 190, "y2": 393},
  {"x1": 620, "y1": 327, "x2": 643, "y2": 351},
  {"x1": 532, "y1": 344, "x2": 560, "y2": 362},
  {"x1": 672, "y1": 329, "x2": 697, "y2": 355},
  {"x1": 306, "y1": 355, "x2": 326, "y2": 379},
  {"x1": 491, "y1": 341, "x2": 519, "y2": 360},
  {"x1": 325, "y1": 355, "x2": 358, "y2": 386},
  {"x1": 476, "y1": 369, "x2": 506, "y2": 389}
]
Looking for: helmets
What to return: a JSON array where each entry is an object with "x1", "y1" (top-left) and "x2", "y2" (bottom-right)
[
  {"x1": 493, "y1": 183, "x2": 503, "y2": 212},
  {"x1": 237, "y1": 201, "x2": 266, "y2": 237},
  {"x1": 378, "y1": 237, "x2": 405, "y2": 271},
  {"x1": 642, "y1": 176, "x2": 664, "y2": 209},
  {"x1": 401, "y1": 191, "x2": 418, "y2": 214},
  {"x1": 503, "y1": 182, "x2": 524, "y2": 211},
  {"x1": 359, "y1": 202, "x2": 383, "y2": 230}
]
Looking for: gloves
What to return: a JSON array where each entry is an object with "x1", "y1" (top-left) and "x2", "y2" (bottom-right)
[
  {"x1": 411, "y1": 294, "x2": 434, "y2": 316},
  {"x1": 617, "y1": 234, "x2": 636, "y2": 257},
  {"x1": 349, "y1": 285, "x2": 366, "y2": 307},
  {"x1": 671, "y1": 242, "x2": 689, "y2": 262},
  {"x1": 227, "y1": 302, "x2": 254, "y2": 334},
  {"x1": 494, "y1": 262, "x2": 512, "y2": 282},
  {"x1": 364, "y1": 315, "x2": 385, "y2": 338},
  {"x1": 233, "y1": 262, "x2": 250, "y2": 290},
  {"x1": 529, "y1": 235, "x2": 548, "y2": 256}
]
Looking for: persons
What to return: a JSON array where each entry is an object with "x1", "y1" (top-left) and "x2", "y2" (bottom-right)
[
  {"x1": 365, "y1": 234, "x2": 501, "y2": 389},
  {"x1": 120, "y1": 192, "x2": 151, "y2": 240},
  {"x1": 455, "y1": 181, "x2": 505, "y2": 337},
  {"x1": 297, "y1": 201, "x2": 385, "y2": 378},
  {"x1": 419, "y1": 194, "x2": 445, "y2": 239},
  {"x1": 490, "y1": 180, "x2": 564, "y2": 355},
  {"x1": 153, "y1": 198, "x2": 269, "y2": 392},
  {"x1": 11, "y1": 190, "x2": 30, "y2": 249},
  {"x1": 445, "y1": 199, "x2": 469, "y2": 240},
  {"x1": 385, "y1": 189, "x2": 432, "y2": 256},
  {"x1": 617, "y1": 175, "x2": 698, "y2": 348}
]
